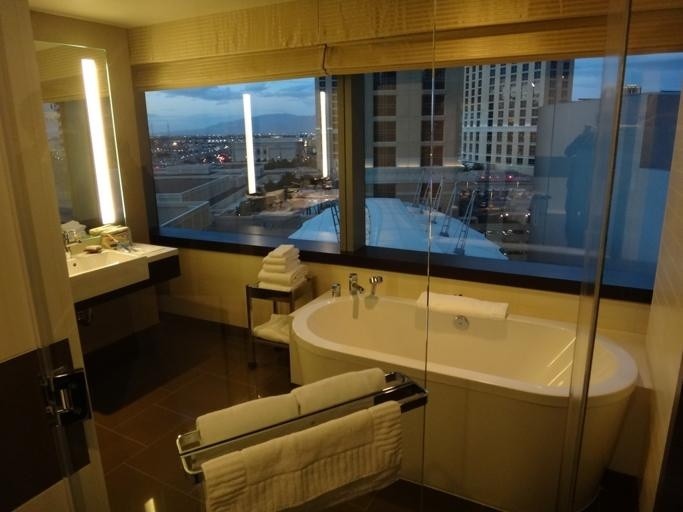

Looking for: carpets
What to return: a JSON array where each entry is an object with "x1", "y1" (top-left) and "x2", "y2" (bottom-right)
[{"x1": 82, "y1": 319, "x2": 197, "y2": 418}]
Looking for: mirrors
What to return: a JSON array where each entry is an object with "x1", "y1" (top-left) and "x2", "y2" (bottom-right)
[{"x1": 31, "y1": 37, "x2": 129, "y2": 231}]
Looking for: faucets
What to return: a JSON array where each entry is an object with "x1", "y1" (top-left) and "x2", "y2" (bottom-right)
[
  {"x1": 63, "y1": 231, "x2": 82, "y2": 258},
  {"x1": 348, "y1": 273, "x2": 364, "y2": 297}
]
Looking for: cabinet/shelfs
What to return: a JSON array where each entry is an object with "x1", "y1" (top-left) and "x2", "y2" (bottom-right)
[{"x1": 245, "y1": 275, "x2": 316, "y2": 370}]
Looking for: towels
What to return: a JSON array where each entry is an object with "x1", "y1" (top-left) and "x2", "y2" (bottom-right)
[
  {"x1": 257, "y1": 245, "x2": 309, "y2": 293},
  {"x1": 196, "y1": 367, "x2": 386, "y2": 464},
  {"x1": 198, "y1": 400, "x2": 402, "y2": 512},
  {"x1": 249, "y1": 312, "x2": 292, "y2": 344}
]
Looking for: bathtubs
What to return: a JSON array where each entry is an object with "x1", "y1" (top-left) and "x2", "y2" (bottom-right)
[{"x1": 291, "y1": 289, "x2": 640, "y2": 512}]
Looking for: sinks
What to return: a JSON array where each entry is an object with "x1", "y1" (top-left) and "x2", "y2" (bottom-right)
[{"x1": 65, "y1": 248, "x2": 151, "y2": 304}]
